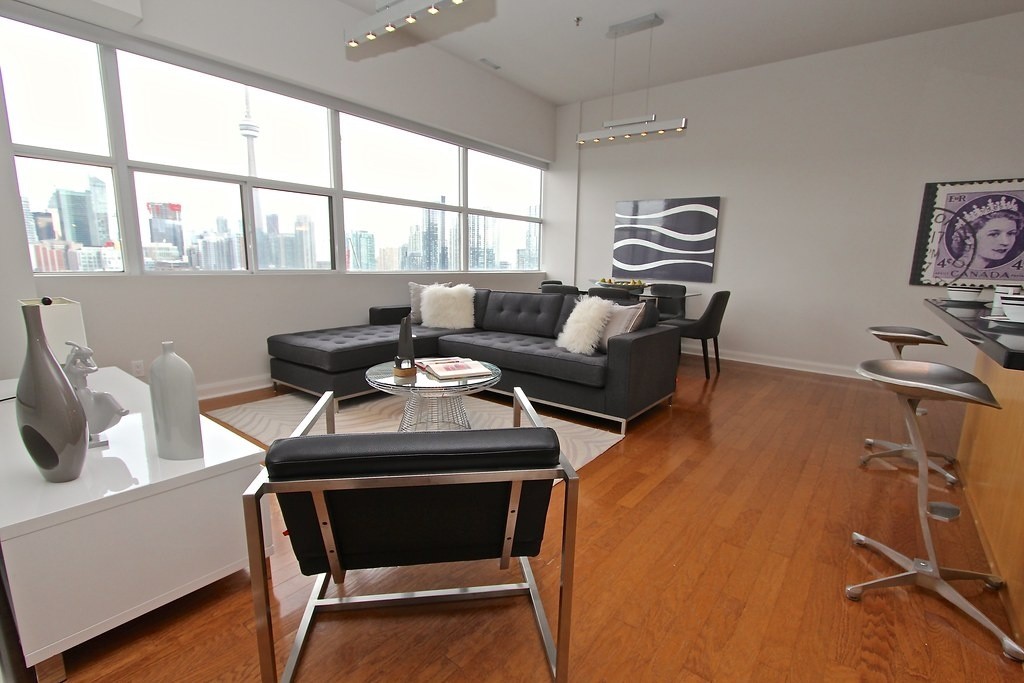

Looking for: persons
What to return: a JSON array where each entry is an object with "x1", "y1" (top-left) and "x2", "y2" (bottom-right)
[
  {"x1": 952, "y1": 196, "x2": 1024, "y2": 269},
  {"x1": 63, "y1": 345, "x2": 129, "y2": 434}
]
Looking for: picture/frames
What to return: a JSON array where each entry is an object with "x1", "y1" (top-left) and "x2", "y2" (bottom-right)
[{"x1": 909, "y1": 178, "x2": 1024, "y2": 294}]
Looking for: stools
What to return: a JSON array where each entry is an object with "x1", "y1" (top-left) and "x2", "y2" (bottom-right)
[
  {"x1": 864, "y1": 322, "x2": 957, "y2": 484},
  {"x1": 845, "y1": 356, "x2": 1023, "y2": 662}
]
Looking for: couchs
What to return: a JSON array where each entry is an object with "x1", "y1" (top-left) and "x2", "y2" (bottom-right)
[{"x1": 262, "y1": 286, "x2": 681, "y2": 435}]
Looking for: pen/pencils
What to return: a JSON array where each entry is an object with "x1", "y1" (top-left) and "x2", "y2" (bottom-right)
[{"x1": 435, "y1": 361, "x2": 459, "y2": 364}]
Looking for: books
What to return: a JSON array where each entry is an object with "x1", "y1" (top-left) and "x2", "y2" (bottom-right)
[{"x1": 416, "y1": 356, "x2": 493, "y2": 379}]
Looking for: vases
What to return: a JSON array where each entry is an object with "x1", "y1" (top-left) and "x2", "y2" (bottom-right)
[
  {"x1": 149, "y1": 341, "x2": 205, "y2": 461},
  {"x1": 16, "y1": 304, "x2": 89, "y2": 483}
]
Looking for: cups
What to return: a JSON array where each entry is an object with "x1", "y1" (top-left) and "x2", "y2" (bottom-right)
[{"x1": 990, "y1": 285, "x2": 1021, "y2": 316}]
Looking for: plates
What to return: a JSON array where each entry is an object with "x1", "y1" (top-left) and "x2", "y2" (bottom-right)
[{"x1": 596, "y1": 281, "x2": 655, "y2": 294}]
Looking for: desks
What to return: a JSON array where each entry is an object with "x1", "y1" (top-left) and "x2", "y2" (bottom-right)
[
  {"x1": 1, "y1": 365, "x2": 264, "y2": 683},
  {"x1": 538, "y1": 286, "x2": 702, "y2": 311}
]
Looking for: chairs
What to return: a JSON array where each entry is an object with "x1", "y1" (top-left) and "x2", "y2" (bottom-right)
[
  {"x1": 655, "y1": 291, "x2": 731, "y2": 379},
  {"x1": 240, "y1": 397, "x2": 580, "y2": 683},
  {"x1": 650, "y1": 284, "x2": 686, "y2": 292},
  {"x1": 587, "y1": 288, "x2": 631, "y2": 304},
  {"x1": 543, "y1": 284, "x2": 577, "y2": 296},
  {"x1": 542, "y1": 280, "x2": 562, "y2": 285}
]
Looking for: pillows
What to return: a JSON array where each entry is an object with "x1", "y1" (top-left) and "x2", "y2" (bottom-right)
[
  {"x1": 405, "y1": 281, "x2": 452, "y2": 322},
  {"x1": 596, "y1": 300, "x2": 647, "y2": 350},
  {"x1": 556, "y1": 296, "x2": 610, "y2": 354},
  {"x1": 418, "y1": 285, "x2": 476, "y2": 329}
]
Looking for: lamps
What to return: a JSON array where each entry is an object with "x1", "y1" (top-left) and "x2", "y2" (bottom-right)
[
  {"x1": 344, "y1": 0, "x2": 463, "y2": 47},
  {"x1": 576, "y1": 12, "x2": 689, "y2": 144}
]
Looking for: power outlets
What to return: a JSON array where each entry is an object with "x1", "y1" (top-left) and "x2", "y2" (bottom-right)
[{"x1": 131, "y1": 361, "x2": 147, "y2": 376}]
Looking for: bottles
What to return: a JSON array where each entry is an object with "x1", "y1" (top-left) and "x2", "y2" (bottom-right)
[
  {"x1": 149, "y1": 342, "x2": 204, "y2": 461},
  {"x1": 16, "y1": 305, "x2": 89, "y2": 483}
]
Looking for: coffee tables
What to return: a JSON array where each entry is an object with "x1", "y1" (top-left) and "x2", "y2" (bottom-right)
[{"x1": 367, "y1": 350, "x2": 501, "y2": 432}]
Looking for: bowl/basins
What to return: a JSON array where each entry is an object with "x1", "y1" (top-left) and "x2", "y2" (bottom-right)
[
  {"x1": 1000, "y1": 295, "x2": 1024, "y2": 321},
  {"x1": 947, "y1": 286, "x2": 983, "y2": 301}
]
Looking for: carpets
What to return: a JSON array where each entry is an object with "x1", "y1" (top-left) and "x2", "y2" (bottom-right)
[{"x1": 206, "y1": 391, "x2": 625, "y2": 485}]
